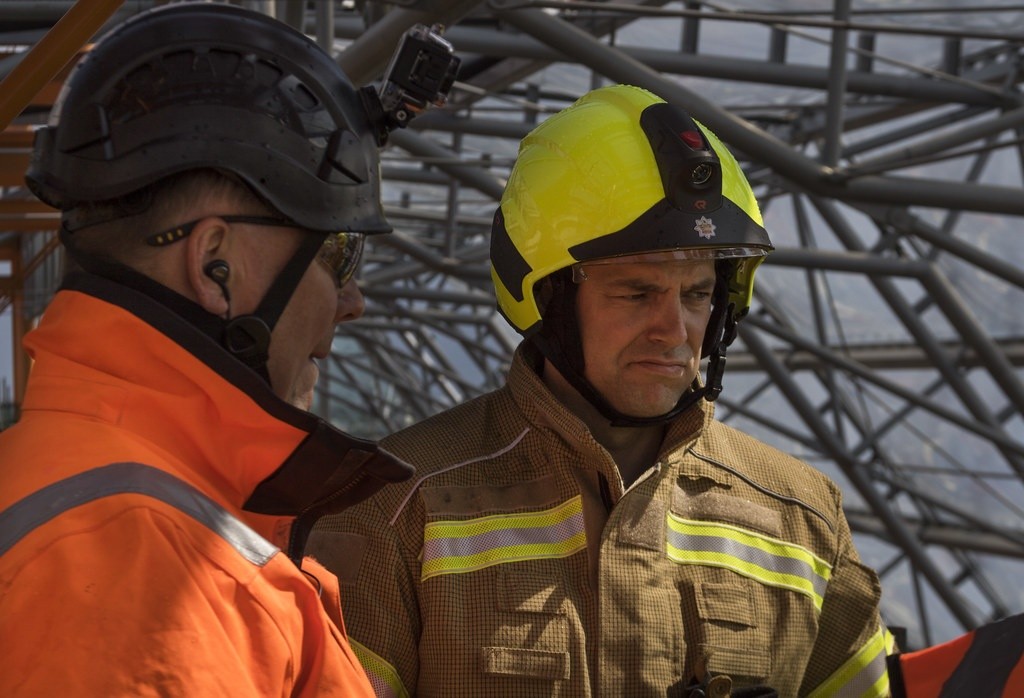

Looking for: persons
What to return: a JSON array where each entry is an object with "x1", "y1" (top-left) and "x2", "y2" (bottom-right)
[
  {"x1": 297, "y1": 82, "x2": 902, "y2": 697},
  {"x1": 0, "y1": 1, "x2": 400, "y2": 698}
]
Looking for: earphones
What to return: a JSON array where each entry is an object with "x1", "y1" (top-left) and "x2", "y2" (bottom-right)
[{"x1": 206, "y1": 259, "x2": 231, "y2": 302}]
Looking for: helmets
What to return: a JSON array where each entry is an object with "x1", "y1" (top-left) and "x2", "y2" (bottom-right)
[
  {"x1": 490, "y1": 84, "x2": 775, "y2": 375},
  {"x1": 24, "y1": 1, "x2": 393, "y2": 233}
]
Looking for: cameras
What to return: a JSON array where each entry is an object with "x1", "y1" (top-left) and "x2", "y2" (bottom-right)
[{"x1": 378, "y1": 23, "x2": 460, "y2": 132}]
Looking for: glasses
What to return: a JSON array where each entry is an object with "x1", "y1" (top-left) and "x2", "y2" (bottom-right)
[{"x1": 148, "y1": 216, "x2": 366, "y2": 289}]
